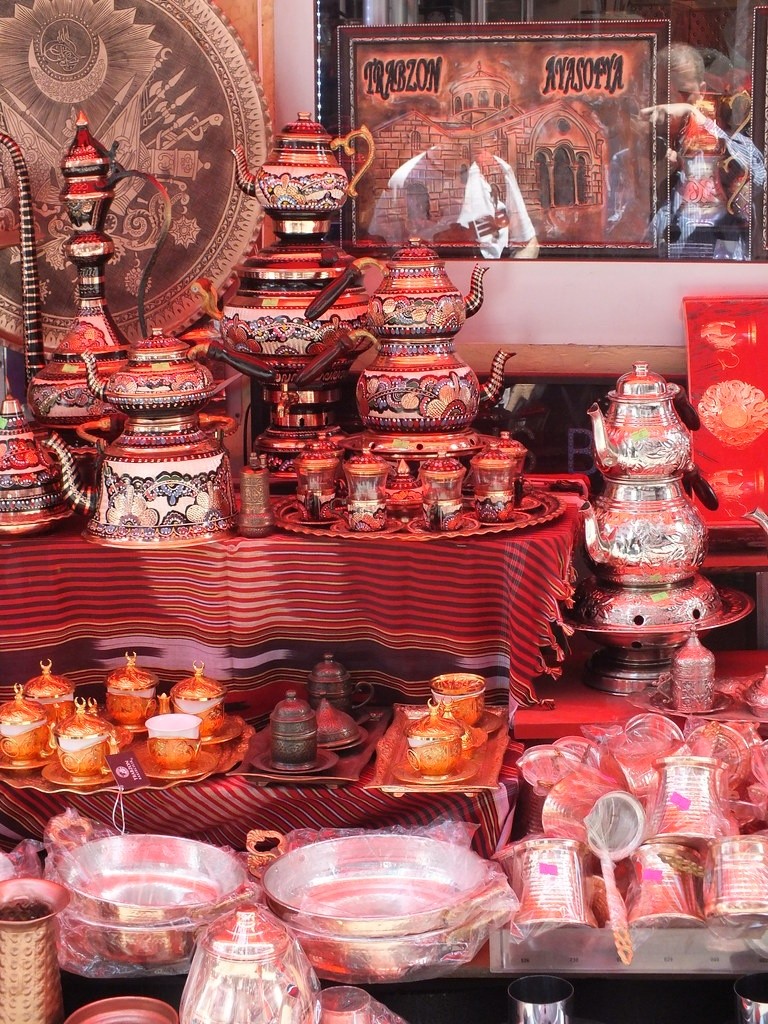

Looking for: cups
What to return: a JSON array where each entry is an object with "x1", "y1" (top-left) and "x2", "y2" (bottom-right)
[
  {"x1": 703, "y1": 835, "x2": 768, "y2": 924},
  {"x1": 628, "y1": 843, "x2": 706, "y2": 928},
  {"x1": 734, "y1": 973, "x2": 768, "y2": 1024},
  {"x1": 320, "y1": 985, "x2": 370, "y2": 1023},
  {"x1": 644, "y1": 756, "x2": 730, "y2": 846},
  {"x1": 518, "y1": 839, "x2": 584, "y2": 928},
  {"x1": 507, "y1": 976, "x2": 572, "y2": 1024},
  {"x1": 300, "y1": 487, "x2": 514, "y2": 531},
  {"x1": 0, "y1": 652, "x2": 488, "y2": 783}
]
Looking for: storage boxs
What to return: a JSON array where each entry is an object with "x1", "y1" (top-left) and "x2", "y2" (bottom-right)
[{"x1": 682, "y1": 295, "x2": 767, "y2": 552}]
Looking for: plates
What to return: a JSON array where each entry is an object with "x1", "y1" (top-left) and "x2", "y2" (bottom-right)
[{"x1": 295, "y1": 496, "x2": 540, "y2": 534}]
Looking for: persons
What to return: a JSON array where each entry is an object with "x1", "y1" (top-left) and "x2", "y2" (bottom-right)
[
  {"x1": 602, "y1": 41, "x2": 767, "y2": 260},
  {"x1": 362, "y1": 115, "x2": 540, "y2": 258}
]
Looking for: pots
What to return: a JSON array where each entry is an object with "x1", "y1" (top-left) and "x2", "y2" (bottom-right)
[{"x1": 45, "y1": 812, "x2": 511, "y2": 977}]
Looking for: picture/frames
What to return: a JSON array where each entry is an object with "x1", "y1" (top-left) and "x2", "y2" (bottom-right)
[{"x1": 330, "y1": 19, "x2": 673, "y2": 259}]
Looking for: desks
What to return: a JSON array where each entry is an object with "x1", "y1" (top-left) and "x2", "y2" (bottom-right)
[
  {"x1": 512, "y1": 648, "x2": 768, "y2": 757},
  {"x1": 0, "y1": 739, "x2": 524, "y2": 860},
  {"x1": 0, "y1": 470, "x2": 593, "y2": 709},
  {"x1": 454, "y1": 339, "x2": 689, "y2": 433}
]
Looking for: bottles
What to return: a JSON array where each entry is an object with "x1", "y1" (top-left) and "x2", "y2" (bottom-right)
[
  {"x1": 296, "y1": 432, "x2": 528, "y2": 502},
  {"x1": 241, "y1": 454, "x2": 273, "y2": 539}
]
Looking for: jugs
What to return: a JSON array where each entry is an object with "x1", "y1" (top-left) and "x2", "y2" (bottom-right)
[
  {"x1": 578, "y1": 458, "x2": 720, "y2": 588},
  {"x1": 81, "y1": 327, "x2": 274, "y2": 417},
  {"x1": 743, "y1": 506, "x2": 768, "y2": 535},
  {"x1": 587, "y1": 362, "x2": 701, "y2": 481},
  {"x1": 229, "y1": 111, "x2": 377, "y2": 242},
  {"x1": 673, "y1": 89, "x2": 750, "y2": 225},
  {"x1": 182, "y1": 900, "x2": 318, "y2": 1024},
  {"x1": 40, "y1": 417, "x2": 238, "y2": 549},
  {"x1": 356, "y1": 339, "x2": 516, "y2": 434},
  {"x1": 304, "y1": 238, "x2": 490, "y2": 337}
]
